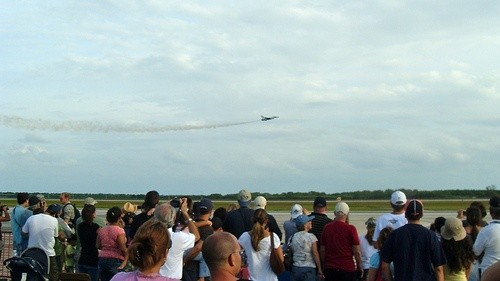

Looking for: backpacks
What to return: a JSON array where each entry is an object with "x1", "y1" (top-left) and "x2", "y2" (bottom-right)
[{"x1": 62, "y1": 203, "x2": 80, "y2": 225}]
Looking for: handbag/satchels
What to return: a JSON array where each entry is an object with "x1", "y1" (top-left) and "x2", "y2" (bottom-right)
[
  {"x1": 269, "y1": 232, "x2": 285, "y2": 274},
  {"x1": 281, "y1": 234, "x2": 294, "y2": 270}
]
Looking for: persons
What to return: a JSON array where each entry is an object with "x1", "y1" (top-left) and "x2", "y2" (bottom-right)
[
  {"x1": 0, "y1": 202, "x2": 11, "y2": 260},
  {"x1": 430, "y1": 192, "x2": 500, "y2": 281},
  {"x1": 10, "y1": 192, "x2": 106, "y2": 281},
  {"x1": 283, "y1": 196, "x2": 364, "y2": 281},
  {"x1": 95, "y1": 190, "x2": 284, "y2": 281},
  {"x1": 357, "y1": 191, "x2": 446, "y2": 281}
]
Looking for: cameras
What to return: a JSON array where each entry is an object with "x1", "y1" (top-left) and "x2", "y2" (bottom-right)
[
  {"x1": 463, "y1": 210, "x2": 467, "y2": 216},
  {"x1": 169, "y1": 197, "x2": 181, "y2": 208}
]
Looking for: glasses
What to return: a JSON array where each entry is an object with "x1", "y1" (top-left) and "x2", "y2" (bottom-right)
[{"x1": 232, "y1": 249, "x2": 245, "y2": 255}]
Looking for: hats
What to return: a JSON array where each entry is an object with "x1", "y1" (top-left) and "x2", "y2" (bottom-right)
[
  {"x1": 313, "y1": 196, "x2": 327, "y2": 207},
  {"x1": 406, "y1": 199, "x2": 423, "y2": 215},
  {"x1": 29, "y1": 196, "x2": 40, "y2": 205},
  {"x1": 296, "y1": 214, "x2": 315, "y2": 227},
  {"x1": 334, "y1": 202, "x2": 350, "y2": 216},
  {"x1": 84, "y1": 197, "x2": 98, "y2": 206},
  {"x1": 196, "y1": 199, "x2": 213, "y2": 213},
  {"x1": 390, "y1": 190, "x2": 407, "y2": 205},
  {"x1": 440, "y1": 216, "x2": 467, "y2": 241},
  {"x1": 237, "y1": 189, "x2": 252, "y2": 202},
  {"x1": 120, "y1": 201, "x2": 138, "y2": 218},
  {"x1": 253, "y1": 196, "x2": 267, "y2": 210},
  {"x1": 47, "y1": 203, "x2": 63, "y2": 214},
  {"x1": 290, "y1": 203, "x2": 303, "y2": 219}
]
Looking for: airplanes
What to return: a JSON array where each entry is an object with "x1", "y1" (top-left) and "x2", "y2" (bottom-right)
[{"x1": 260, "y1": 114, "x2": 281, "y2": 121}]
